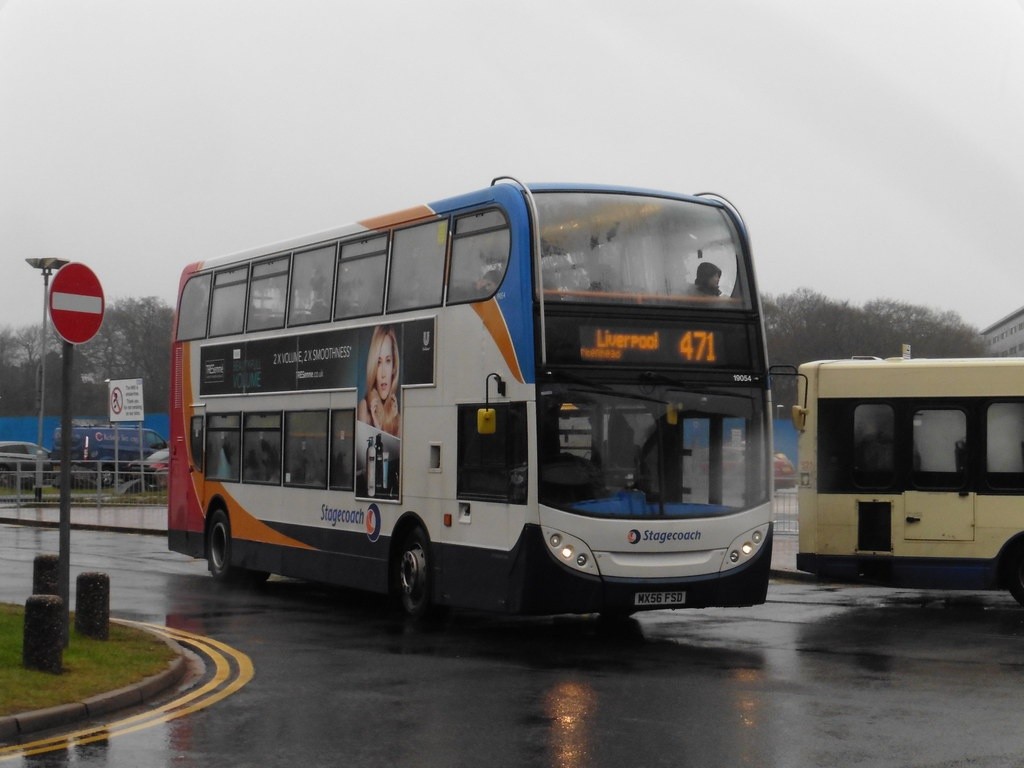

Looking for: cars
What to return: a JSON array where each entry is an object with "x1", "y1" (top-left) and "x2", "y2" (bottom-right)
[
  {"x1": 123, "y1": 447, "x2": 170, "y2": 487},
  {"x1": 684, "y1": 445, "x2": 796, "y2": 492},
  {"x1": 0, "y1": 441, "x2": 54, "y2": 490}
]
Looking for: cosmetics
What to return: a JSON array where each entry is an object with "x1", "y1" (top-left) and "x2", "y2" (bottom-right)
[{"x1": 364, "y1": 433, "x2": 389, "y2": 496}]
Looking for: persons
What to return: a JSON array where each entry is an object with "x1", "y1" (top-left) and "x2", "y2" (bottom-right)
[
  {"x1": 690, "y1": 263, "x2": 722, "y2": 296},
  {"x1": 357, "y1": 325, "x2": 401, "y2": 439}
]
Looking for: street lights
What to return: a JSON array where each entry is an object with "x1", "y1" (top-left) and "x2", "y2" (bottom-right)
[{"x1": 25, "y1": 258, "x2": 70, "y2": 503}]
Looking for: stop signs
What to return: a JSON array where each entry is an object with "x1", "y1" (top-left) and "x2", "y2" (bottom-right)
[{"x1": 49, "y1": 263, "x2": 105, "y2": 344}]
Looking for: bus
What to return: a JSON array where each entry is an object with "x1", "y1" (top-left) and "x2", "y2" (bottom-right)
[
  {"x1": 796, "y1": 357, "x2": 1023, "y2": 610},
  {"x1": 169, "y1": 176, "x2": 808, "y2": 635}
]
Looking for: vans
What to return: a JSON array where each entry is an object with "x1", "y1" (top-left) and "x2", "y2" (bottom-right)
[{"x1": 52, "y1": 426, "x2": 167, "y2": 490}]
